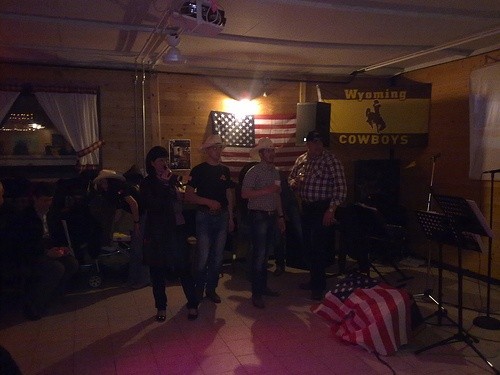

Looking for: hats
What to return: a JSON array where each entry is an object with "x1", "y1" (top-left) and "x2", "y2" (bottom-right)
[
  {"x1": 250, "y1": 138, "x2": 281, "y2": 160},
  {"x1": 199, "y1": 134, "x2": 226, "y2": 152},
  {"x1": 303, "y1": 131, "x2": 326, "y2": 142},
  {"x1": 93, "y1": 169, "x2": 126, "y2": 184}
]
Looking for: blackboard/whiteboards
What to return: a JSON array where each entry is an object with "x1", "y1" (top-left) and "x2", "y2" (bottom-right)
[{"x1": 467, "y1": 60, "x2": 500, "y2": 183}]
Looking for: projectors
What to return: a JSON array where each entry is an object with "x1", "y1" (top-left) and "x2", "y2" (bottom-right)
[{"x1": 176, "y1": 2, "x2": 226, "y2": 35}]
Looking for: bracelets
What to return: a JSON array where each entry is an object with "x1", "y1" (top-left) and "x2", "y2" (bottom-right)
[
  {"x1": 279, "y1": 216, "x2": 283, "y2": 217},
  {"x1": 134, "y1": 220, "x2": 141, "y2": 224}
]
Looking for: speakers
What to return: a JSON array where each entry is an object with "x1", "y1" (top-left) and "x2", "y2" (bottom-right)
[{"x1": 296, "y1": 102, "x2": 330, "y2": 147}]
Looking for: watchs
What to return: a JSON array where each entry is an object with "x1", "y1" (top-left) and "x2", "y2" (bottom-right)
[{"x1": 326, "y1": 208, "x2": 335, "y2": 212}]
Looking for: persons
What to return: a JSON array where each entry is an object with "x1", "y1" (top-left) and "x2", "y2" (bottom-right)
[{"x1": 9, "y1": 129, "x2": 347, "y2": 321}]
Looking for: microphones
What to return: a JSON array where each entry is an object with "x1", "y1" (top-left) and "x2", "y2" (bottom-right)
[{"x1": 167, "y1": 170, "x2": 183, "y2": 189}]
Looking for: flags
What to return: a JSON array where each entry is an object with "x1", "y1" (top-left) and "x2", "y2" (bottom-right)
[
  {"x1": 314, "y1": 270, "x2": 411, "y2": 355},
  {"x1": 212, "y1": 112, "x2": 302, "y2": 174}
]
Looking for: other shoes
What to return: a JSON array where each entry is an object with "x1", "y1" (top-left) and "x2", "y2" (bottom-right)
[
  {"x1": 274, "y1": 264, "x2": 285, "y2": 276},
  {"x1": 157, "y1": 304, "x2": 167, "y2": 321},
  {"x1": 205, "y1": 290, "x2": 221, "y2": 303},
  {"x1": 190, "y1": 308, "x2": 198, "y2": 318},
  {"x1": 252, "y1": 298, "x2": 265, "y2": 308},
  {"x1": 300, "y1": 280, "x2": 312, "y2": 290},
  {"x1": 312, "y1": 288, "x2": 321, "y2": 299}
]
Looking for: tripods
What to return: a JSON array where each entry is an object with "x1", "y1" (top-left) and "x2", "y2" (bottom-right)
[{"x1": 415, "y1": 193, "x2": 500, "y2": 374}]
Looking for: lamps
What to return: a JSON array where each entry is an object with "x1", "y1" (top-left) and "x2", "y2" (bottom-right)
[{"x1": 161, "y1": 34, "x2": 186, "y2": 65}]
